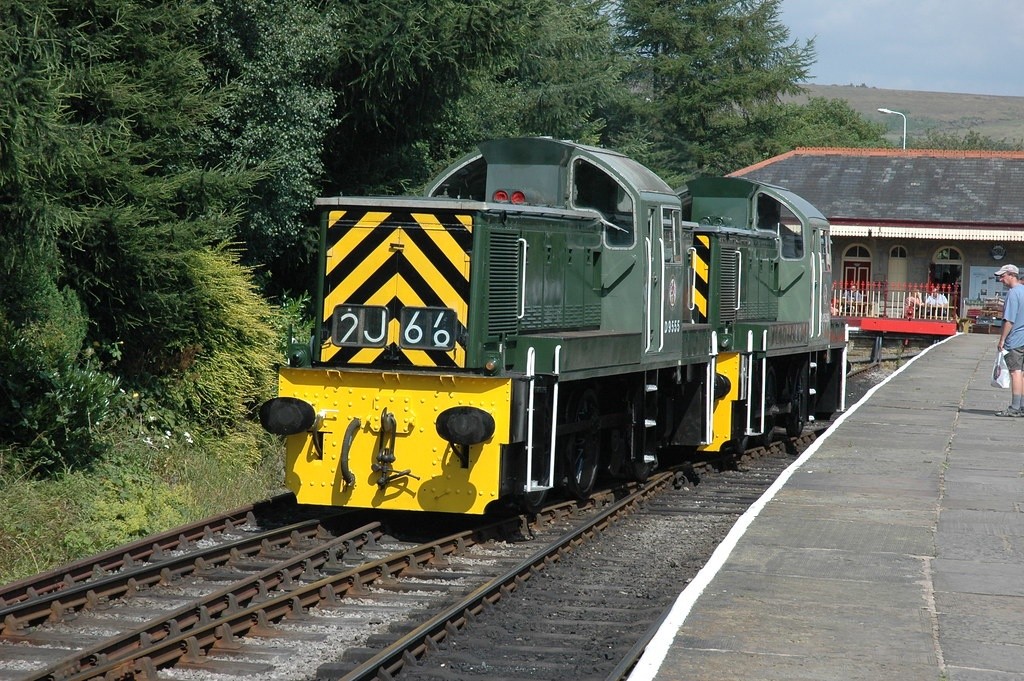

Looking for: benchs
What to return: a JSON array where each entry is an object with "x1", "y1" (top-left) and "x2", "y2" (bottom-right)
[
  {"x1": 833, "y1": 311, "x2": 867, "y2": 317},
  {"x1": 914, "y1": 314, "x2": 960, "y2": 332}
]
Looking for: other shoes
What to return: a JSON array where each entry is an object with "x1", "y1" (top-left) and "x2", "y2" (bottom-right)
[{"x1": 994, "y1": 406, "x2": 1024, "y2": 417}]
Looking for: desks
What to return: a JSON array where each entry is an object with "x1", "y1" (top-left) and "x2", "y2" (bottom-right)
[
  {"x1": 918, "y1": 304, "x2": 957, "y2": 316},
  {"x1": 836, "y1": 300, "x2": 868, "y2": 317}
]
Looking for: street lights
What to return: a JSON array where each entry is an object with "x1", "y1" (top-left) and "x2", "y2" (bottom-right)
[{"x1": 876, "y1": 107, "x2": 907, "y2": 150}]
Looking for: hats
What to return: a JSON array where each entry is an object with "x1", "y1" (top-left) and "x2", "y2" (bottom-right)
[{"x1": 994, "y1": 264, "x2": 1019, "y2": 276}]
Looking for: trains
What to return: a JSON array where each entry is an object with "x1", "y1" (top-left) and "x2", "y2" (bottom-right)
[{"x1": 259, "y1": 129, "x2": 852, "y2": 519}]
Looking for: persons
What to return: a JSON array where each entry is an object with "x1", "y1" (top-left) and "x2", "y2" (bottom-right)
[
  {"x1": 993, "y1": 264, "x2": 1024, "y2": 417},
  {"x1": 831, "y1": 296, "x2": 840, "y2": 316},
  {"x1": 905, "y1": 290, "x2": 920, "y2": 319},
  {"x1": 843, "y1": 284, "x2": 862, "y2": 301},
  {"x1": 925, "y1": 287, "x2": 948, "y2": 319}
]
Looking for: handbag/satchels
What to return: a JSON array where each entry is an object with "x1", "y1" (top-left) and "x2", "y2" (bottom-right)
[{"x1": 990, "y1": 347, "x2": 1010, "y2": 389}]
